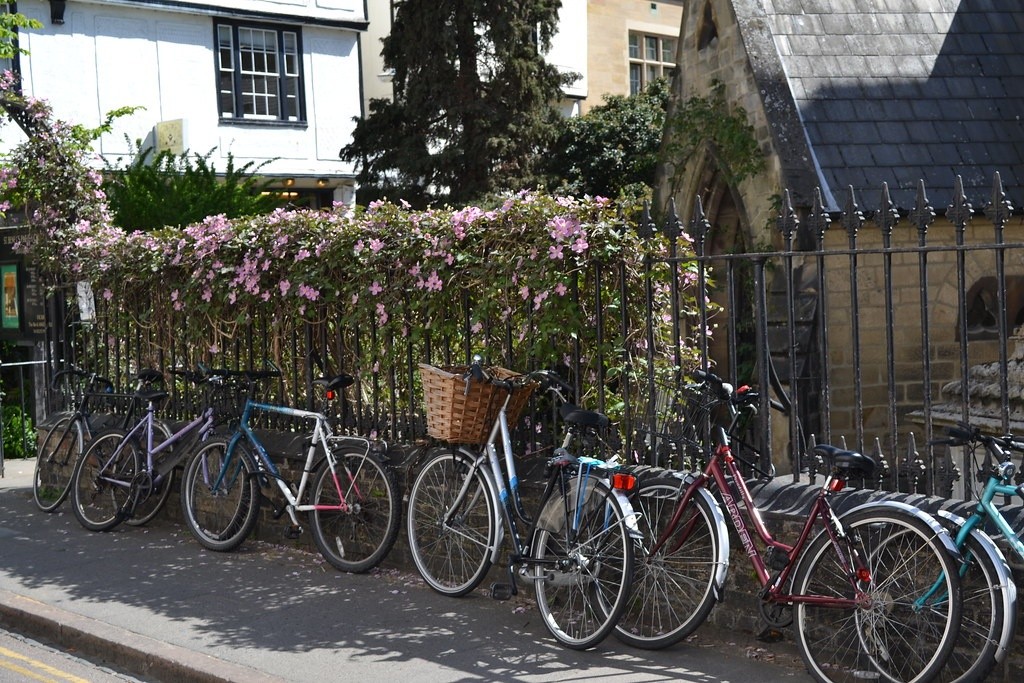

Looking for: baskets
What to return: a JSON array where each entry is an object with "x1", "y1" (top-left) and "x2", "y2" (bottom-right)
[
  {"x1": 636, "y1": 375, "x2": 716, "y2": 445},
  {"x1": 417, "y1": 362, "x2": 538, "y2": 445}
]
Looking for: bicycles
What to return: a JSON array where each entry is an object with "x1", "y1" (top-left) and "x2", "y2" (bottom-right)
[
  {"x1": 588, "y1": 367, "x2": 965, "y2": 683},
  {"x1": 846, "y1": 422, "x2": 1024, "y2": 683},
  {"x1": 69, "y1": 368, "x2": 250, "y2": 542},
  {"x1": 179, "y1": 357, "x2": 407, "y2": 575},
  {"x1": 403, "y1": 350, "x2": 647, "y2": 652},
  {"x1": 33, "y1": 368, "x2": 177, "y2": 527}
]
